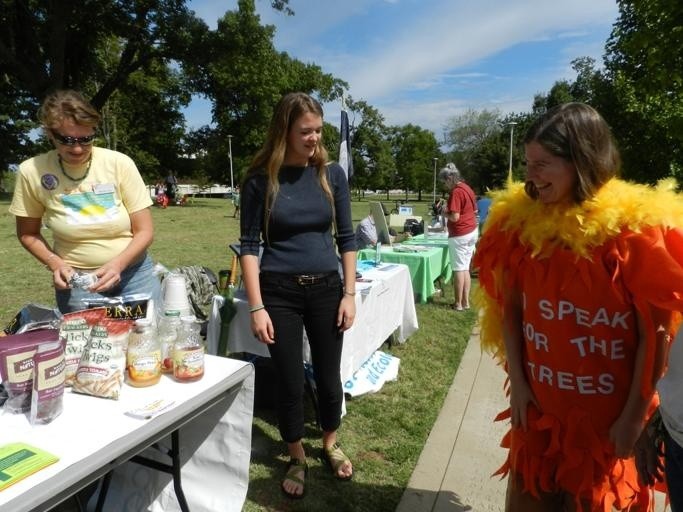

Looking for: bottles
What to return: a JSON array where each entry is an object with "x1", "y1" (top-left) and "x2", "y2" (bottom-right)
[
  {"x1": 126, "y1": 319, "x2": 161, "y2": 387},
  {"x1": 157, "y1": 311, "x2": 180, "y2": 373},
  {"x1": 174, "y1": 314, "x2": 206, "y2": 381}
]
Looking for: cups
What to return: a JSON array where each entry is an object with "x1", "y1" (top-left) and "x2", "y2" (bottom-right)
[{"x1": 166, "y1": 277, "x2": 190, "y2": 320}]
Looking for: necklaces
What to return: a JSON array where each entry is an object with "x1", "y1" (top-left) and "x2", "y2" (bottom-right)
[{"x1": 58, "y1": 151, "x2": 92, "y2": 182}]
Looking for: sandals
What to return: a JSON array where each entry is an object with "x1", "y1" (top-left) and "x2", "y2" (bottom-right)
[
  {"x1": 322, "y1": 440, "x2": 354, "y2": 482},
  {"x1": 281, "y1": 459, "x2": 309, "y2": 500}
]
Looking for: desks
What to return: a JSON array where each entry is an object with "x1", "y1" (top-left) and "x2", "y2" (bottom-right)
[
  {"x1": 358, "y1": 245, "x2": 451, "y2": 304},
  {"x1": 0, "y1": 353, "x2": 256, "y2": 512},
  {"x1": 406, "y1": 232, "x2": 455, "y2": 285},
  {"x1": 206, "y1": 263, "x2": 420, "y2": 435}
]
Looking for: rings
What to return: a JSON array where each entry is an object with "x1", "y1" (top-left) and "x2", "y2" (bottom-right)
[
  {"x1": 111, "y1": 285, "x2": 115, "y2": 289},
  {"x1": 254, "y1": 334, "x2": 259, "y2": 340}
]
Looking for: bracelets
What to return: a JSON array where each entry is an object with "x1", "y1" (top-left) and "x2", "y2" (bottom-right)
[
  {"x1": 344, "y1": 290, "x2": 356, "y2": 297},
  {"x1": 248, "y1": 304, "x2": 264, "y2": 313},
  {"x1": 45, "y1": 253, "x2": 55, "y2": 270}
]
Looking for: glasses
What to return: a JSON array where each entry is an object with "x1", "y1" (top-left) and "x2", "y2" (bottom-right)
[{"x1": 49, "y1": 127, "x2": 98, "y2": 147}]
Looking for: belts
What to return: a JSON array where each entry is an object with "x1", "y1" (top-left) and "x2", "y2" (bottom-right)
[{"x1": 288, "y1": 272, "x2": 339, "y2": 286}]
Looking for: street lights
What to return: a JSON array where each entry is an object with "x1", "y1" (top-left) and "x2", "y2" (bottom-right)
[
  {"x1": 431, "y1": 156, "x2": 439, "y2": 204},
  {"x1": 226, "y1": 134, "x2": 235, "y2": 190},
  {"x1": 507, "y1": 121, "x2": 517, "y2": 177}
]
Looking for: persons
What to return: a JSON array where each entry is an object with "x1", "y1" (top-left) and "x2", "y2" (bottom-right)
[
  {"x1": 354, "y1": 202, "x2": 412, "y2": 250},
  {"x1": 432, "y1": 162, "x2": 478, "y2": 311},
  {"x1": 469, "y1": 102, "x2": 683, "y2": 511},
  {"x1": 151, "y1": 168, "x2": 187, "y2": 208},
  {"x1": 634, "y1": 324, "x2": 683, "y2": 511},
  {"x1": 8, "y1": 90, "x2": 162, "y2": 322},
  {"x1": 239, "y1": 92, "x2": 358, "y2": 500},
  {"x1": 231, "y1": 187, "x2": 240, "y2": 219}
]
